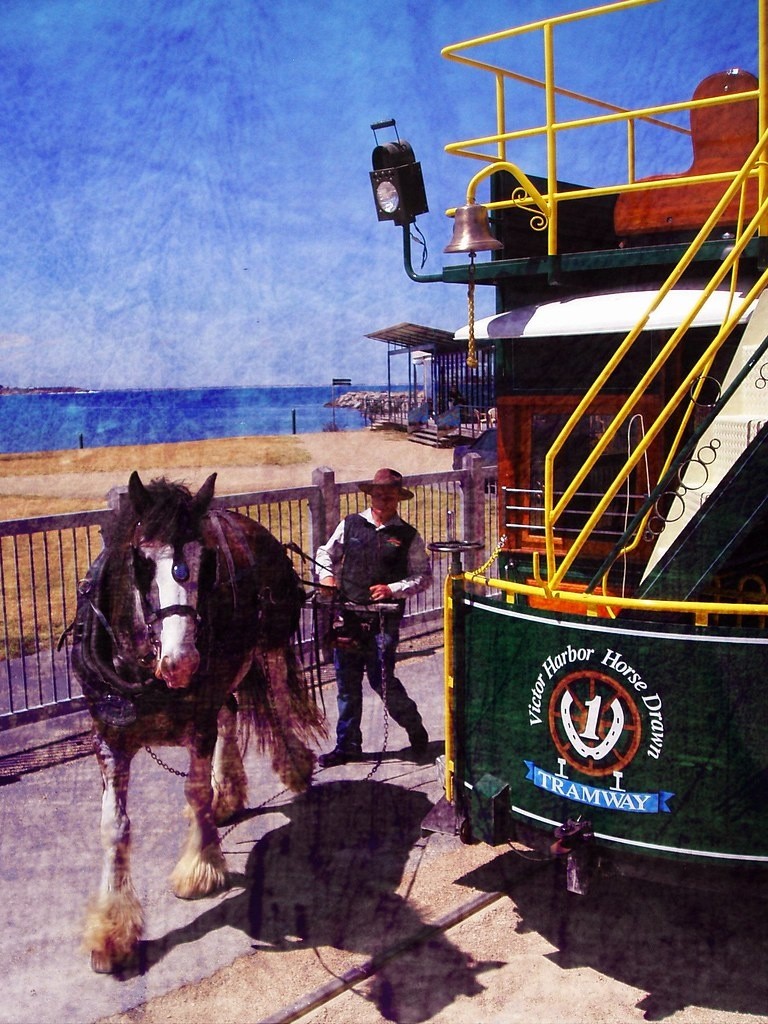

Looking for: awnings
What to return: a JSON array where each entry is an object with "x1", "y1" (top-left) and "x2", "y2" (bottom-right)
[{"x1": 363, "y1": 322, "x2": 456, "y2": 356}]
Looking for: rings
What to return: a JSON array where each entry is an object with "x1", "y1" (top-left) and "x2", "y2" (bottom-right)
[{"x1": 378, "y1": 588, "x2": 383, "y2": 590}]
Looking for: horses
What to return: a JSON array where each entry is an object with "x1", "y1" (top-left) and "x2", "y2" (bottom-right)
[{"x1": 69, "y1": 470, "x2": 331, "y2": 976}]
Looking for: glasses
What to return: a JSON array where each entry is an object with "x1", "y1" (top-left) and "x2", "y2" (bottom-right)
[{"x1": 370, "y1": 493, "x2": 396, "y2": 503}]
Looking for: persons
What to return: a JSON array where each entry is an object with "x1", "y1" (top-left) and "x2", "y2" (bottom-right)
[
  {"x1": 315, "y1": 468, "x2": 434, "y2": 768},
  {"x1": 448, "y1": 384, "x2": 463, "y2": 409}
]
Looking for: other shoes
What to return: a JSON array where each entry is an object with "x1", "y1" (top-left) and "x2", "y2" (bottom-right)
[
  {"x1": 318, "y1": 744, "x2": 364, "y2": 767},
  {"x1": 408, "y1": 727, "x2": 429, "y2": 762}
]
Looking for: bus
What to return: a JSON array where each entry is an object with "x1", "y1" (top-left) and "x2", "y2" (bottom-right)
[{"x1": 356, "y1": 0, "x2": 768, "y2": 910}]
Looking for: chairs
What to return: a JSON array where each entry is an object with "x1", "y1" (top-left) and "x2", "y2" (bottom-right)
[{"x1": 614, "y1": 68, "x2": 758, "y2": 242}]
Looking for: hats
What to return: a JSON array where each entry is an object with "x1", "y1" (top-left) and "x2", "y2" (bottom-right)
[{"x1": 356, "y1": 468, "x2": 415, "y2": 500}]
